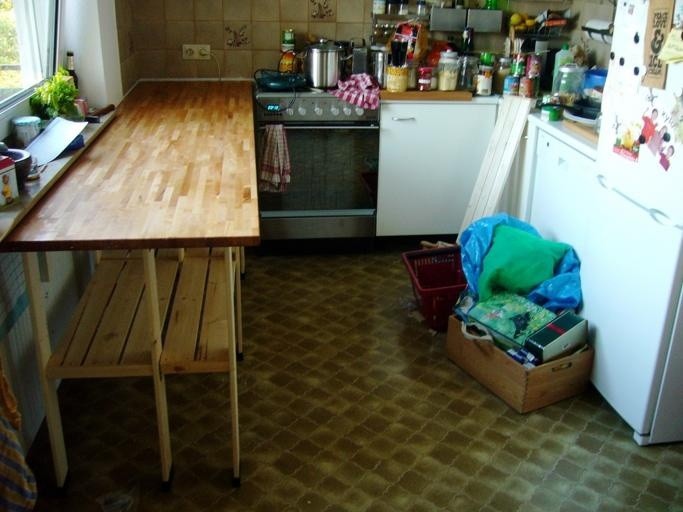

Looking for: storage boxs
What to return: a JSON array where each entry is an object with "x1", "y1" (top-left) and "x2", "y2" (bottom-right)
[{"x1": 446, "y1": 313, "x2": 593, "y2": 416}]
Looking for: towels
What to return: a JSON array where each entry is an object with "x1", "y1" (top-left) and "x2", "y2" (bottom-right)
[
  {"x1": 0, "y1": 414, "x2": 39, "y2": 512},
  {"x1": 0, "y1": 363, "x2": 22, "y2": 430},
  {"x1": 257, "y1": 124, "x2": 291, "y2": 194},
  {"x1": 326, "y1": 73, "x2": 380, "y2": 110}
]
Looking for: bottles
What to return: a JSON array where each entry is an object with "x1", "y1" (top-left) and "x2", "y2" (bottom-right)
[
  {"x1": 391, "y1": 39, "x2": 407, "y2": 65},
  {"x1": 555, "y1": 64, "x2": 584, "y2": 98},
  {"x1": 370, "y1": 50, "x2": 383, "y2": 88},
  {"x1": 387, "y1": 1, "x2": 398, "y2": 14},
  {"x1": 477, "y1": 65, "x2": 492, "y2": 95},
  {"x1": 484, "y1": 0, "x2": 497, "y2": 11},
  {"x1": 453, "y1": 0, "x2": 463, "y2": 9},
  {"x1": 417, "y1": 1, "x2": 426, "y2": 16},
  {"x1": 372, "y1": 1, "x2": 386, "y2": 15},
  {"x1": 438, "y1": 52, "x2": 458, "y2": 90},
  {"x1": 65, "y1": 50, "x2": 78, "y2": 90},
  {"x1": 399, "y1": 0, "x2": 409, "y2": 15},
  {"x1": 495, "y1": 58, "x2": 510, "y2": 92},
  {"x1": 552, "y1": 43, "x2": 574, "y2": 94},
  {"x1": 353, "y1": 37, "x2": 369, "y2": 75},
  {"x1": 459, "y1": 57, "x2": 475, "y2": 89}
]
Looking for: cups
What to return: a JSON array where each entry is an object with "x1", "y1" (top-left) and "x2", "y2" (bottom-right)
[{"x1": 536, "y1": 42, "x2": 548, "y2": 65}]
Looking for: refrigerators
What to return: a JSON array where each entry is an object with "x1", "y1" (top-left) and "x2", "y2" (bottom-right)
[{"x1": 581, "y1": 0, "x2": 682, "y2": 446}]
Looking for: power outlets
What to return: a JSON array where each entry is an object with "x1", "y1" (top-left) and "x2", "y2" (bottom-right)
[{"x1": 183, "y1": 44, "x2": 210, "y2": 61}]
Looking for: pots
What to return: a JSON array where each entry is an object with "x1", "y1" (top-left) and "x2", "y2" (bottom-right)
[
  {"x1": 259, "y1": 67, "x2": 307, "y2": 93},
  {"x1": 306, "y1": 38, "x2": 352, "y2": 88}
]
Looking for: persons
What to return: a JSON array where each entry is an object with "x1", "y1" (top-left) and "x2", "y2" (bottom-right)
[{"x1": 641, "y1": 107, "x2": 674, "y2": 171}]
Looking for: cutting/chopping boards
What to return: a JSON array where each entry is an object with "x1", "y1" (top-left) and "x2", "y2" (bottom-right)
[{"x1": 379, "y1": 87, "x2": 472, "y2": 102}]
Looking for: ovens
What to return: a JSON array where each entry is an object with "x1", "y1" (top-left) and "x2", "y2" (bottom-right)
[{"x1": 256, "y1": 124, "x2": 377, "y2": 214}]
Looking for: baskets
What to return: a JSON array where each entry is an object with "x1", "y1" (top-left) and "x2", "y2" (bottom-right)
[{"x1": 400, "y1": 245, "x2": 468, "y2": 334}]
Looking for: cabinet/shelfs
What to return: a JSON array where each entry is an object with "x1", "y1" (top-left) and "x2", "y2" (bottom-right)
[
  {"x1": 0, "y1": 81, "x2": 261, "y2": 491},
  {"x1": 528, "y1": 131, "x2": 596, "y2": 246},
  {"x1": 375, "y1": 100, "x2": 501, "y2": 237}
]
